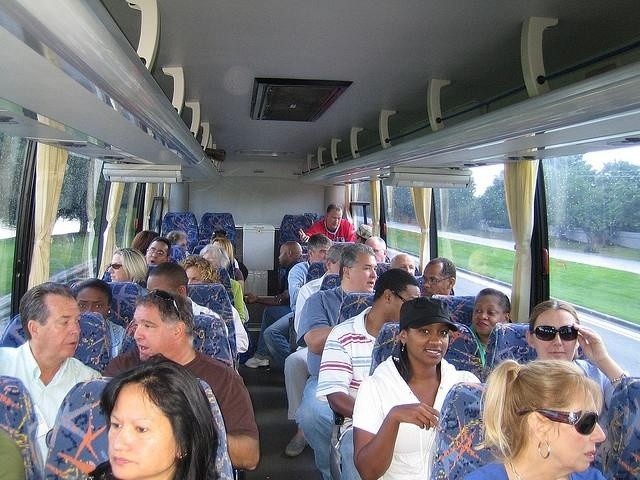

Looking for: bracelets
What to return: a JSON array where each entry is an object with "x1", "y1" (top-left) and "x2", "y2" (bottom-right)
[{"x1": 609, "y1": 373, "x2": 628, "y2": 383}]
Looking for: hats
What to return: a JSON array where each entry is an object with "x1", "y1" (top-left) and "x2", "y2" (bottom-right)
[
  {"x1": 398, "y1": 296, "x2": 461, "y2": 333},
  {"x1": 352, "y1": 223, "x2": 373, "y2": 240}
]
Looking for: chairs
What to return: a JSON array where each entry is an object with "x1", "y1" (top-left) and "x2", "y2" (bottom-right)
[
  {"x1": 0, "y1": 374, "x2": 44, "y2": 479},
  {"x1": 215, "y1": 266, "x2": 236, "y2": 308},
  {"x1": 187, "y1": 239, "x2": 197, "y2": 252},
  {"x1": 189, "y1": 284, "x2": 237, "y2": 363},
  {"x1": 429, "y1": 381, "x2": 494, "y2": 480},
  {"x1": 416, "y1": 276, "x2": 455, "y2": 295},
  {"x1": 161, "y1": 212, "x2": 197, "y2": 239},
  {"x1": 101, "y1": 263, "x2": 158, "y2": 288},
  {"x1": 228, "y1": 263, "x2": 235, "y2": 277},
  {"x1": 334, "y1": 293, "x2": 374, "y2": 426},
  {"x1": 486, "y1": 322, "x2": 594, "y2": 371},
  {"x1": 201, "y1": 239, "x2": 237, "y2": 249},
  {"x1": 43, "y1": 377, "x2": 234, "y2": 480},
  {"x1": 589, "y1": 377, "x2": 640, "y2": 479},
  {"x1": 331, "y1": 241, "x2": 354, "y2": 248},
  {"x1": 307, "y1": 262, "x2": 326, "y2": 283},
  {"x1": 69, "y1": 281, "x2": 139, "y2": 326},
  {"x1": 373, "y1": 263, "x2": 419, "y2": 291},
  {"x1": 369, "y1": 322, "x2": 485, "y2": 383},
  {"x1": 119, "y1": 315, "x2": 235, "y2": 367},
  {"x1": 320, "y1": 273, "x2": 340, "y2": 292},
  {"x1": 432, "y1": 296, "x2": 477, "y2": 321},
  {"x1": 168, "y1": 246, "x2": 186, "y2": 263},
  {"x1": 193, "y1": 245, "x2": 203, "y2": 255},
  {"x1": 279, "y1": 247, "x2": 307, "y2": 282},
  {"x1": 0, "y1": 312, "x2": 113, "y2": 376},
  {"x1": 198, "y1": 213, "x2": 237, "y2": 238},
  {"x1": 277, "y1": 216, "x2": 309, "y2": 245}
]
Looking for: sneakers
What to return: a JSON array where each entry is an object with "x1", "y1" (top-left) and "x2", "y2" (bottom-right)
[
  {"x1": 284, "y1": 434, "x2": 310, "y2": 457},
  {"x1": 244, "y1": 356, "x2": 270, "y2": 369}
]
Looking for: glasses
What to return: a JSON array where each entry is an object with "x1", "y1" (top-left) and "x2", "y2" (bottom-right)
[
  {"x1": 527, "y1": 325, "x2": 581, "y2": 342},
  {"x1": 146, "y1": 247, "x2": 167, "y2": 259},
  {"x1": 423, "y1": 274, "x2": 451, "y2": 286},
  {"x1": 151, "y1": 290, "x2": 182, "y2": 320},
  {"x1": 422, "y1": 274, "x2": 450, "y2": 285},
  {"x1": 393, "y1": 288, "x2": 410, "y2": 304},
  {"x1": 106, "y1": 263, "x2": 124, "y2": 269},
  {"x1": 518, "y1": 405, "x2": 606, "y2": 434}
]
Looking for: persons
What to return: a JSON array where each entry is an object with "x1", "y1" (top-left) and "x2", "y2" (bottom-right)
[
  {"x1": 147, "y1": 261, "x2": 228, "y2": 335},
  {"x1": 352, "y1": 297, "x2": 481, "y2": 479},
  {"x1": 349, "y1": 223, "x2": 372, "y2": 242},
  {"x1": 298, "y1": 203, "x2": 355, "y2": 243},
  {"x1": 365, "y1": 235, "x2": 389, "y2": 266},
  {"x1": 210, "y1": 237, "x2": 240, "y2": 271},
  {"x1": 166, "y1": 230, "x2": 190, "y2": 252},
  {"x1": 108, "y1": 249, "x2": 146, "y2": 283},
  {"x1": 471, "y1": 288, "x2": 512, "y2": 338},
  {"x1": 0, "y1": 282, "x2": 100, "y2": 462},
  {"x1": 126, "y1": 229, "x2": 157, "y2": 252},
  {"x1": 392, "y1": 254, "x2": 418, "y2": 277},
  {"x1": 105, "y1": 290, "x2": 259, "y2": 471},
  {"x1": 100, "y1": 354, "x2": 220, "y2": 480},
  {"x1": 297, "y1": 243, "x2": 377, "y2": 480},
  {"x1": 285, "y1": 242, "x2": 350, "y2": 459},
  {"x1": 423, "y1": 258, "x2": 457, "y2": 296},
  {"x1": 144, "y1": 236, "x2": 171, "y2": 266},
  {"x1": 181, "y1": 255, "x2": 249, "y2": 353},
  {"x1": 464, "y1": 360, "x2": 603, "y2": 480},
  {"x1": 525, "y1": 299, "x2": 629, "y2": 382},
  {"x1": 315, "y1": 268, "x2": 421, "y2": 480},
  {"x1": 200, "y1": 244, "x2": 250, "y2": 323},
  {"x1": 71, "y1": 279, "x2": 126, "y2": 360}
]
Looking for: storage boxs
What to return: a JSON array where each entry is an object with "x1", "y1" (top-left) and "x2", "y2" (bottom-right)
[
  {"x1": 243, "y1": 271, "x2": 268, "y2": 298},
  {"x1": 242, "y1": 224, "x2": 274, "y2": 270}
]
